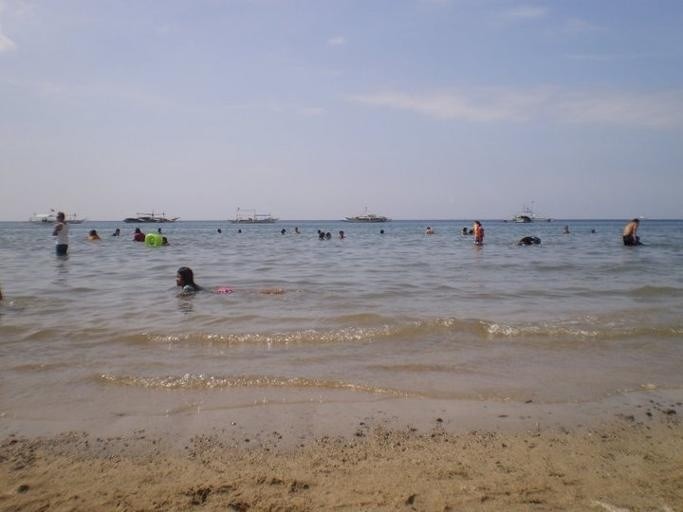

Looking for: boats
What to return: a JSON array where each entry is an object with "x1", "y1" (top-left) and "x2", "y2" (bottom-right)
[
  {"x1": 31, "y1": 208, "x2": 85, "y2": 223},
  {"x1": 504, "y1": 212, "x2": 559, "y2": 223},
  {"x1": 122, "y1": 213, "x2": 180, "y2": 222},
  {"x1": 227, "y1": 208, "x2": 279, "y2": 224},
  {"x1": 344, "y1": 213, "x2": 392, "y2": 222}
]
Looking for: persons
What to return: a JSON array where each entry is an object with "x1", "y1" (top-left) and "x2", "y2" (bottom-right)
[
  {"x1": 425, "y1": 226, "x2": 432, "y2": 236},
  {"x1": 52, "y1": 212, "x2": 68, "y2": 256},
  {"x1": 295, "y1": 227, "x2": 300, "y2": 233},
  {"x1": 317, "y1": 230, "x2": 331, "y2": 240},
  {"x1": 112, "y1": 228, "x2": 120, "y2": 236},
  {"x1": 463, "y1": 220, "x2": 484, "y2": 244},
  {"x1": 87, "y1": 229, "x2": 100, "y2": 240},
  {"x1": 337, "y1": 231, "x2": 347, "y2": 240},
  {"x1": 281, "y1": 229, "x2": 286, "y2": 235},
  {"x1": 563, "y1": 224, "x2": 571, "y2": 234},
  {"x1": 135, "y1": 228, "x2": 170, "y2": 246},
  {"x1": 623, "y1": 219, "x2": 642, "y2": 246},
  {"x1": 175, "y1": 267, "x2": 285, "y2": 297}
]
